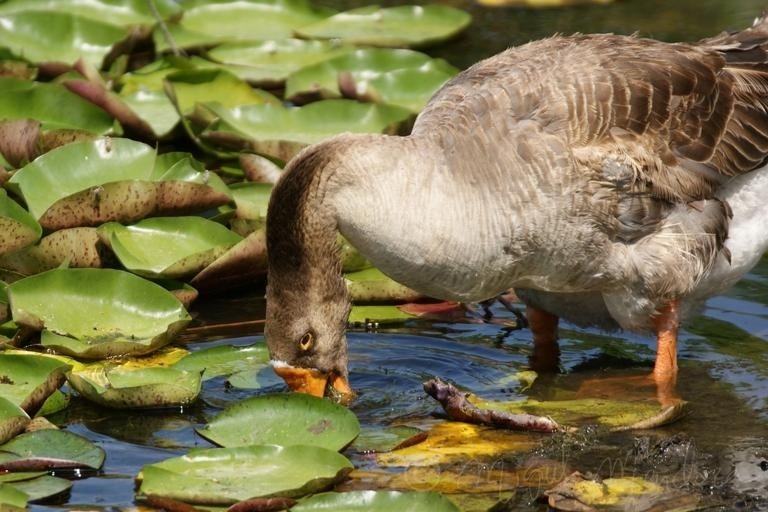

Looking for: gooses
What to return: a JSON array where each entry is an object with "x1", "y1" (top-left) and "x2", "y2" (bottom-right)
[{"x1": 263, "y1": 7, "x2": 768, "y2": 411}]
